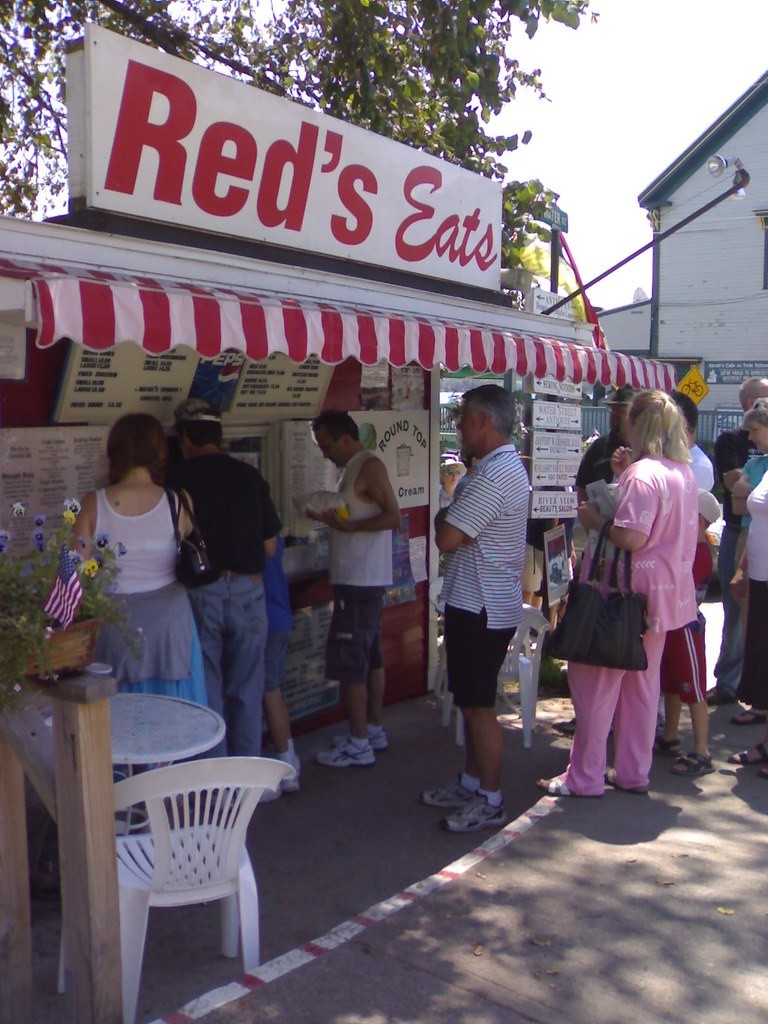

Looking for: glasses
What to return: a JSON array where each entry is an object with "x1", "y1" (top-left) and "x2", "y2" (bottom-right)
[{"x1": 606, "y1": 405, "x2": 629, "y2": 414}]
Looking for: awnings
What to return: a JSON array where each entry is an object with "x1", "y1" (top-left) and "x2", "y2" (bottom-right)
[{"x1": 0, "y1": 257, "x2": 678, "y2": 391}]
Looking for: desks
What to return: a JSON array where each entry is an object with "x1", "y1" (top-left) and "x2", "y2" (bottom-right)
[{"x1": 42, "y1": 691, "x2": 227, "y2": 835}]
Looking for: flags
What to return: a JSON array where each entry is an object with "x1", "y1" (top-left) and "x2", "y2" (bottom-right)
[{"x1": 42, "y1": 543, "x2": 83, "y2": 631}]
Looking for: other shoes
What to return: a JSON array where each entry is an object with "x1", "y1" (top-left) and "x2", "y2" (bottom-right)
[
  {"x1": 190, "y1": 791, "x2": 234, "y2": 811},
  {"x1": 276, "y1": 755, "x2": 300, "y2": 790},
  {"x1": 234, "y1": 786, "x2": 283, "y2": 804}
]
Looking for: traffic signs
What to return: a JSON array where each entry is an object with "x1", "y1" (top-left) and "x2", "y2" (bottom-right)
[
  {"x1": 532, "y1": 461, "x2": 580, "y2": 486},
  {"x1": 533, "y1": 373, "x2": 583, "y2": 401},
  {"x1": 530, "y1": 491, "x2": 580, "y2": 519},
  {"x1": 532, "y1": 431, "x2": 583, "y2": 462},
  {"x1": 533, "y1": 400, "x2": 582, "y2": 432}
]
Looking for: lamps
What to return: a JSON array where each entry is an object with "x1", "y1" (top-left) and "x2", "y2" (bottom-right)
[
  {"x1": 703, "y1": 154, "x2": 742, "y2": 176},
  {"x1": 731, "y1": 187, "x2": 744, "y2": 198}
]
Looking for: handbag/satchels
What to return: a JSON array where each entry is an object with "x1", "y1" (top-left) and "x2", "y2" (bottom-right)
[
  {"x1": 544, "y1": 519, "x2": 648, "y2": 671},
  {"x1": 165, "y1": 485, "x2": 219, "y2": 588}
]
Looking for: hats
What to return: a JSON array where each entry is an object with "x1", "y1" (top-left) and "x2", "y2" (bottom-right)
[
  {"x1": 174, "y1": 399, "x2": 210, "y2": 420},
  {"x1": 601, "y1": 383, "x2": 646, "y2": 405},
  {"x1": 698, "y1": 489, "x2": 720, "y2": 524}
]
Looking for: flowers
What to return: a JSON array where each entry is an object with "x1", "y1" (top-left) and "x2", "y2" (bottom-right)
[{"x1": 0, "y1": 496, "x2": 149, "y2": 702}]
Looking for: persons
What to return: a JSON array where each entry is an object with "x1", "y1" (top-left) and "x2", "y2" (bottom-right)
[
  {"x1": 310, "y1": 410, "x2": 402, "y2": 769},
  {"x1": 517, "y1": 486, "x2": 577, "y2": 631},
  {"x1": 653, "y1": 490, "x2": 720, "y2": 777},
  {"x1": 727, "y1": 471, "x2": 768, "y2": 780},
  {"x1": 422, "y1": 384, "x2": 529, "y2": 833},
  {"x1": 531, "y1": 389, "x2": 715, "y2": 797},
  {"x1": 731, "y1": 396, "x2": 768, "y2": 725},
  {"x1": 68, "y1": 414, "x2": 207, "y2": 782},
  {"x1": 707, "y1": 378, "x2": 768, "y2": 705},
  {"x1": 167, "y1": 399, "x2": 302, "y2": 810}
]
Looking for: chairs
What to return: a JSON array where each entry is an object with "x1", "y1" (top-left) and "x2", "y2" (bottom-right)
[
  {"x1": 428, "y1": 577, "x2": 552, "y2": 749},
  {"x1": 51, "y1": 753, "x2": 297, "y2": 1024}
]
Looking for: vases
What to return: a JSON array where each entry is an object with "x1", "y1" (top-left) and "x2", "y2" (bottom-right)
[{"x1": 0, "y1": 617, "x2": 101, "y2": 682}]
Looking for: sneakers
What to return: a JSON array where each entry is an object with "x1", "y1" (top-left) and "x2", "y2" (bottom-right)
[
  {"x1": 419, "y1": 773, "x2": 478, "y2": 808},
  {"x1": 332, "y1": 727, "x2": 388, "y2": 750},
  {"x1": 440, "y1": 788, "x2": 507, "y2": 832},
  {"x1": 316, "y1": 736, "x2": 376, "y2": 767}
]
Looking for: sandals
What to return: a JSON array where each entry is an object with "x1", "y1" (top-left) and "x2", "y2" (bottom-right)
[
  {"x1": 604, "y1": 766, "x2": 650, "y2": 794},
  {"x1": 728, "y1": 741, "x2": 768, "y2": 764},
  {"x1": 535, "y1": 775, "x2": 605, "y2": 798},
  {"x1": 671, "y1": 748, "x2": 715, "y2": 776},
  {"x1": 758, "y1": 766, "x2": 768, "y2": 776},
  {"x1": 705, "y1": 686, "x2": 739, "y2": 706},
  {"x1": 651, "y1": 734, "x2": 682, "y2": 757},
  {"x1": 732, "y1": 707, "x2": 768, "y2": 725}
]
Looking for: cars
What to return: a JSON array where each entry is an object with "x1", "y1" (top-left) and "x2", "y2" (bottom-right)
[{"x1": 571, "y1": 504, "x2": 726, "y2": 587}]
